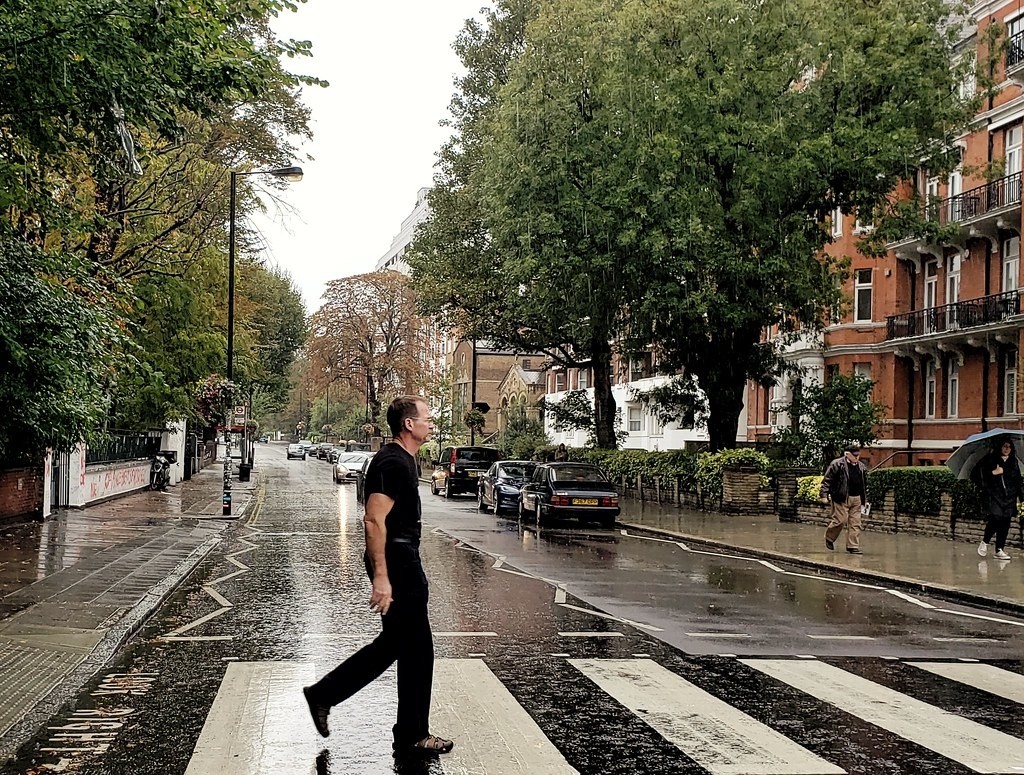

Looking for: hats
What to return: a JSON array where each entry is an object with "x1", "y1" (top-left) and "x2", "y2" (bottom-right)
[{"x1": 844, "y1": 444, "x2": 860, "y2": 455}]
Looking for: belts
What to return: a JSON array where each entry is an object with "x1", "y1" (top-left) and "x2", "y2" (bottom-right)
[{"x1": 387, "y1": 536, "x2": 417, "y2": 543}]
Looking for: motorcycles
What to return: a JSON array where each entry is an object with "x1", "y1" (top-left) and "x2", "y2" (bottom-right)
[{"x1": 150, "y1": 447, "x2": 177, "y2": 491}]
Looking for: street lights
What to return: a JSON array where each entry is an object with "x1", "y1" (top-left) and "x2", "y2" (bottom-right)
[{"x1": 221, "y1": 164, "x2": 304, "y2": 518}]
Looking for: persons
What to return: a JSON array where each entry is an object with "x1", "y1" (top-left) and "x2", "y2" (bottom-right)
[
  {"x1": 302, "y1": 394, "x2": 454, "y2": 755},
  {"x1": 968, "y1": 436, "x2": 1024, "y2": 559},
  {"x1": 817, "y1": 444, "x2": 868, "y2": 554},
  {"x1": 554, "y1": 443, "x2": 569, "y2": 462}
]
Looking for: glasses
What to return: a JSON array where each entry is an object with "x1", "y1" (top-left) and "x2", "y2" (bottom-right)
[{"x1": 405, "y1": 416, "x2": 434, "y2": 423}]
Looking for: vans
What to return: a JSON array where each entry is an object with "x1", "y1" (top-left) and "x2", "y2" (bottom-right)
[{"x1": 430, "y1": 446, "x2": 502, "y2": 499}]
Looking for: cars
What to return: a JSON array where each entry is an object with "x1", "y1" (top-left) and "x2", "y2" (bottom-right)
[
  {"x1": 258, "y1": 435, "x2": 268, "y2": 444},
  {"x1": 475, "y1": 459, "x2": 542, "y2": 517},
  {"x1": 297, "y1": 439, "x2": 313, "y2": 453},
  {"x1": 308, "y1": 441, "x2": 378, "y2": 484},
  {"x1": 516, "y1": 461, "x2": 621, "y2": 529},
  {"x1": 285, "y1": 443, "x2": 306, "y2": 460}
]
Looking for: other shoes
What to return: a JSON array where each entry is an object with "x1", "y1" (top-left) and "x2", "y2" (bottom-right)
[
  {"x1": 993, "y1": 548, "x2": 1010, "y2": 559},
  {"x1": 825, "y1": 539, "x2": 834, "y2": 550},
  {"x1": 978, "y1": 540, "x2": 987, "y2": 556},
  {"x1": 303, "y1": 686, "x2": 331, "y2": 738},
  {"x1": 392, "y1": 734, "x2": 454, "y2": 752},
  {"x1": 846, "y1": 548, "x2": 862, "y2": 554}
]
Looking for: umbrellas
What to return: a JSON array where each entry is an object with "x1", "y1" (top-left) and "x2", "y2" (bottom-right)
[{"x1": 943, "y1": 427, "x2": 1024, "y2": 495}]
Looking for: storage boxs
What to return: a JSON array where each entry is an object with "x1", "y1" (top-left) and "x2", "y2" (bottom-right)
[{"x1": 158, "y1": 450, "x2": 177, "y2": 464}]
